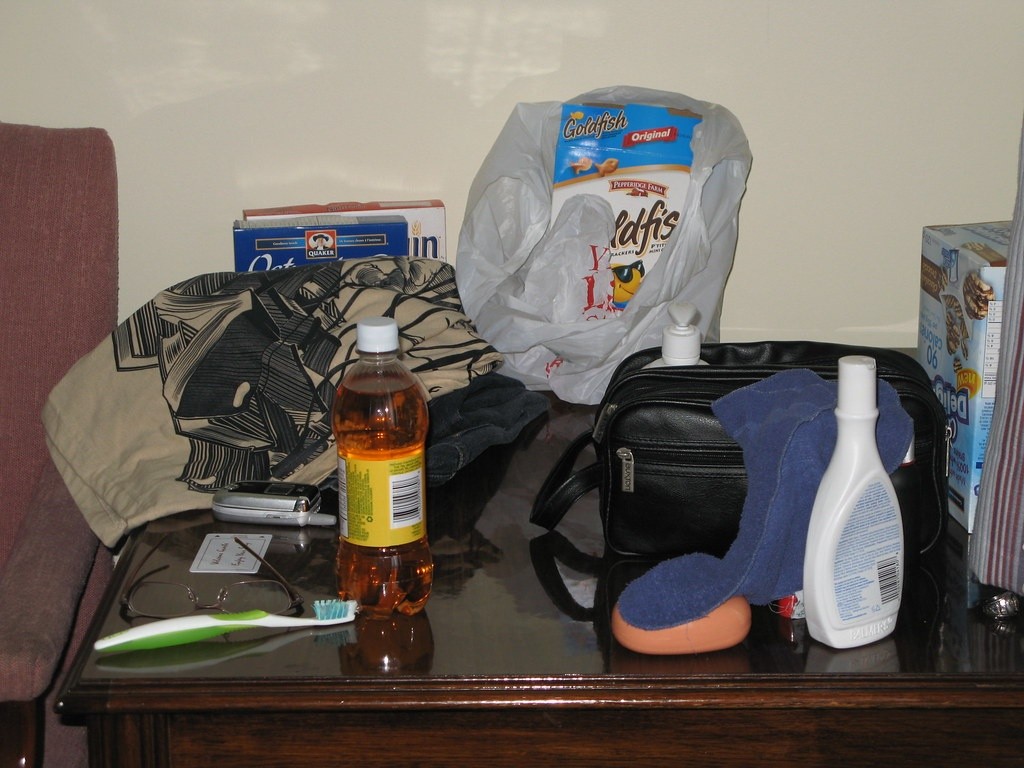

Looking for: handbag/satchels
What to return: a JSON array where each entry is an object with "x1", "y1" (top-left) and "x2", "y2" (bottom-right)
[
  {"x1": 456, "y1": 85, "x2": 754, "y2": 406},
  {"x1": 530, "y1": 340, "x2": 957, "y2": 565}
]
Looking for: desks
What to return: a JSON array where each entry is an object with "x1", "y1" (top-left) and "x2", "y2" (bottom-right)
[{"x1": 55, "y1": 439, "x2": 1024, "y2": 768}]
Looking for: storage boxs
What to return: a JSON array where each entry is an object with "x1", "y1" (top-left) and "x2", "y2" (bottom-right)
[
  {"x1": 242, "y1": 198, "x2": 447, "y2": 263},
  {"x1": 549, "y1": 100, "x2": 703, "y2": 311},
  {"x1": 233, "y1": 214, "x2": 408, "y2": 271},
  {"x1": 917, "y1": 220, "x2": 1013, "y2": 533}
]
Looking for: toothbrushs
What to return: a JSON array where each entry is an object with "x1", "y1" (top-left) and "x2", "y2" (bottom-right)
[{"x1": 91, "y1": 595, "x2": 359, "y2": 658}]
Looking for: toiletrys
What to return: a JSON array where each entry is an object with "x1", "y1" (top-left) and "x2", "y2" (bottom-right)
[{"x1": 801, "y1": 352, "x2": 908, "y2": 653}]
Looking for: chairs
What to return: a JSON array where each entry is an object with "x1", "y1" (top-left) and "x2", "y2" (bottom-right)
[{"x1": 0, "y1": 121, "x2": 121, "y2": 768}]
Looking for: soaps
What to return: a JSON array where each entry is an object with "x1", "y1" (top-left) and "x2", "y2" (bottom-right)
[{"x1": 608, "y1": 594, "x2": 753, "y2": 657}]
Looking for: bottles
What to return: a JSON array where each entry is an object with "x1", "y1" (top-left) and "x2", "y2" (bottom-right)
[{"x1": 331, "y1": 319, "x2": 435, "y2": 615}]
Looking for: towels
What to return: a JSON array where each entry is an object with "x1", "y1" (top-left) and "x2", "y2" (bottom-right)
[{"x1": 613, "y1": 365, "x2": 920, "y2": 631}]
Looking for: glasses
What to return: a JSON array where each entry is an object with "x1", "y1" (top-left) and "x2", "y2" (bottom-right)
[{"x1": 119, "y1": 531, "x2": 307, "y2": 618}]
